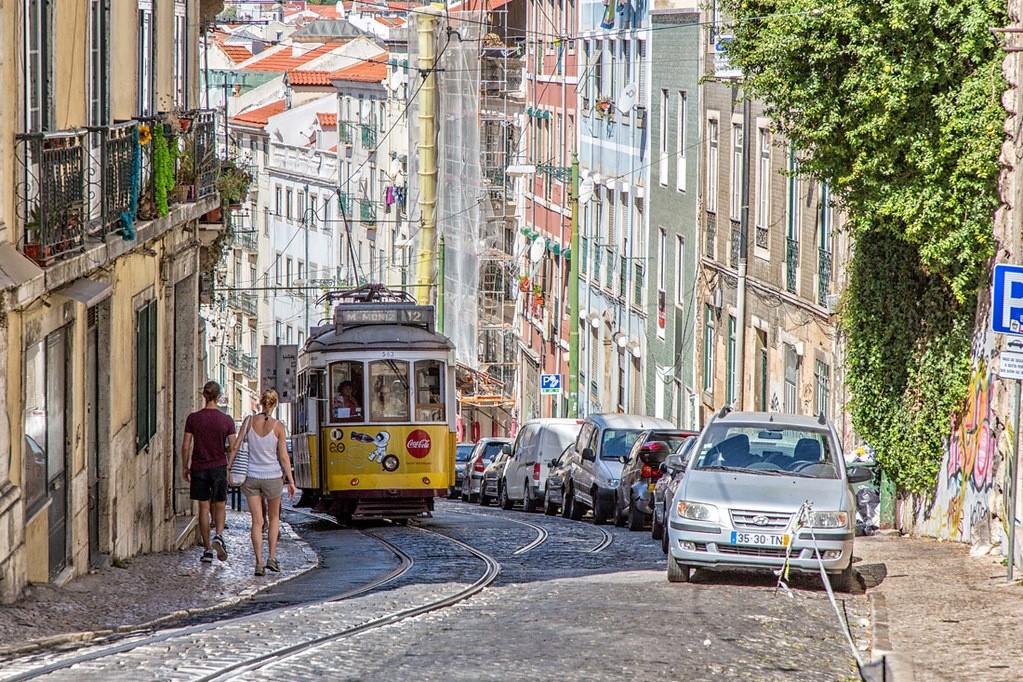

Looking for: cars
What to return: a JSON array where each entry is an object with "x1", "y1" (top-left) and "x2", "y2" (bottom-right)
[
  {"x1": 478, "y1": 443, "x2": 512, "y2": 506},
  {"x1": 543, "y1": 443, "x2": 578, "y2": 518},
  {"x1": 446, "y1": 444, "x2": 476, "y2": 498},
  {"x1": 653, "y1": 436, "x2": 787, "y2": 557},
  {"x1": 613, "y1": 429, "x2": 704, "y2": 531},
  {"x1": 462, "y1": 436, "x2": 517, "y2": 503}
]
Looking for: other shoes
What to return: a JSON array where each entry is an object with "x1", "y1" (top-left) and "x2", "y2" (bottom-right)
[
  {"x1": 262, "y1": 523, "x2": 269, "y2": 532},
  {"x1": 210, "y1": 522, "x2": 215, "y2": 528},
  {"x1": 265, "y1": 559, "x2": 280, "y2": 572},
  {"x1": 255, "y1": 565, "x2": 265, "y2": 576},
  {"x1": 212, "y1": 537, "x2": 227, "y2": 562},
  {"x1": 200, "y1": 551, "x2": 213, "y2": 562},
  {"x1": 224, "y1": 524, "x2": 229, "y2": 529}
]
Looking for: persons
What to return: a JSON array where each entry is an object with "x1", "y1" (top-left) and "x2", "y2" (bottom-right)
[
  {"x1": 182, "y1": 381, "x2": 236, "y2": 563},
  {"x1": 334, "y1": 380, "x2": 360, "y2": 415},
  {"x1": 372, "y1": 385, "x2": 404, "y2": 414},
  {"x1": 210, "y1": 489, "x2": 229, "y2": 529},
  {"x1": 260, "y1": 443, "x2": 288, "y2": 537},
  {"x1": 226, "y1": 390, "x2": 296, "y2": 575}
]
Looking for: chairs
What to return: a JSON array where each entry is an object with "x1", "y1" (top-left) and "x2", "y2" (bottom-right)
[{"x1": 793, "y1": 439, "x2": 820, "y2": 473}]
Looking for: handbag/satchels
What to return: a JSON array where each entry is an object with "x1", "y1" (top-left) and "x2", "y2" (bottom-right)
[{"x1": 229, "y1": 416, "x2": 253, "y2": 486}]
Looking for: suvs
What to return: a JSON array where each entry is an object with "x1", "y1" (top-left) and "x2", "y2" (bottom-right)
[{"x1": 666, "y1": 406, "x2": 873, "y2": 592}]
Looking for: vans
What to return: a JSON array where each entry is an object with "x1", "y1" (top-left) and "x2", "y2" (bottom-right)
[
  {"x1": 500, "y1": 418, "x2": 585, "y2": 512},
  {"x1": 568, "y1": 413, "x2": 680, "y2": 525}
]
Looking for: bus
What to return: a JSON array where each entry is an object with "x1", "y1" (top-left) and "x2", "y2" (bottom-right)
[{"x1": 289, "y1": 282, "x2": 460, "y2": 525}]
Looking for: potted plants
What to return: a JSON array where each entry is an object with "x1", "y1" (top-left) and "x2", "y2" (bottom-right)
[
  {"x1": 26, "y1": 200, "x2": 87, "y2": 258},
  {"x1": 172, "y1": 172, "x2": 195, "y2": 202}
]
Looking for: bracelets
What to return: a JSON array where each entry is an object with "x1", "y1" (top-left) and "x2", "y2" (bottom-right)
[{"x1": 227, "y1": 468, "x2": 231, "y2": 471}]
